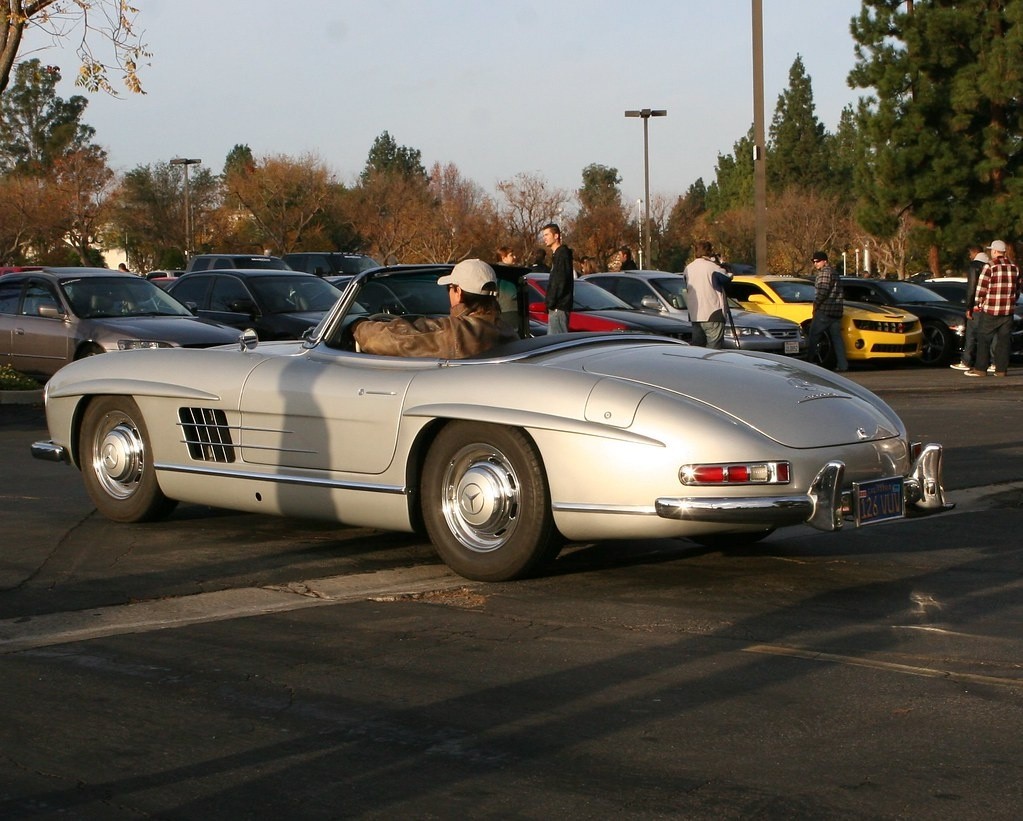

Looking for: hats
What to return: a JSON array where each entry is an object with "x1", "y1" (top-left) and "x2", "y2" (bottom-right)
[
  {"x1": 437, "y1": 259, "x2": 498, "y2": 296},
  {"x1": 987, "y1": 241, "x2": 1006, "y2": 251},
  {"x1": 813, "y1": 251, "x2": 827, "y2": 260}
]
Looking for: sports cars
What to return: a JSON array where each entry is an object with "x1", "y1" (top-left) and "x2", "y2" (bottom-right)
[
  {"x1": 32, "y1": 258, "x2": 959, "y2": 584},
  {"x1": 0, "y1": 267, "x2": 245, "y2": 387}
]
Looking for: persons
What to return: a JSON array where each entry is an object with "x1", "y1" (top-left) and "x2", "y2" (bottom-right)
[
  {"x1": 950, "y1": 246, "x2": 990, "y2": 370},
  {"x1": 618, "y1": 247, "x2": 642, "y2": 305},
  {"x1": 576, "y1": 256, "x2": 606, "y2": 291},
  {"x1": 683, "y1": 240, "x2": 733, "y2": 350},
  {"x1": 529, "y1": 248, "x2": 551, "y2": 273},
  {"x1": 543, "y1": 224, "x2": 574, "y2": 335},
  {"x1": 806, "y1": 252, "x2": 848, "y2": 372},
  {"x1": 496, "y1": 246, "x2": 518, "y2": 332},
  {"x1": 963, "y1": 240, "x2": 1022, "y2": 377},
  {"x1": 344, "y1": 259, "x2": 520, "y2": 358},
  {"x1": 118, "y1": 263, "x2": 137, "y2": 313},
  {"x1": 861, "y1": 271, "x2": 881, "y2": 301}
]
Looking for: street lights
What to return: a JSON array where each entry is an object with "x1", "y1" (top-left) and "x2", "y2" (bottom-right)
[
  {"x1": 170, "y1": 158, "x2": 201, "y2": 268},
  {"x1": 625, "y1": 108, "x2": 667, "y2": 269}
]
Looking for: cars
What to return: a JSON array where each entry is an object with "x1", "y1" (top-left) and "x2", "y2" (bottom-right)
[
  {"x1": 159, "y1": 236, "x2": 1022, "y2": 372},
  {"x1": 134, "y1": 268, "x2": 371, "y2": 342}
]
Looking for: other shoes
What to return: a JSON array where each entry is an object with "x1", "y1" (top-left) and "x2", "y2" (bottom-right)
[
  {"x1": 994, "y1": 371, "x2": 1007, "y2": 376},
  {"x1": 964, "y1": 368, "x2": 987, "y2": 377},
  {"x1": 987, "y1": 364, "x2": 996, "y2": 372},
  {"x1": 950, "y1": 363, "x2": 970, "y2": 370},
  {"x1": 832, "y1": 364, "x2": 848, "y2": 371}
]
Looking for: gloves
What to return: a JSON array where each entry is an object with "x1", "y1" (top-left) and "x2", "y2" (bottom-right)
[{"x1": 351, "y1": 318, "x2": 370, "y2": 335}]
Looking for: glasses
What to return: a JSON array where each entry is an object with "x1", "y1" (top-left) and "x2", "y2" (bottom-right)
[
  {"x1": 813, "y1": 260, "x2": 821, "y2": 263},
  {"x1": 447, "y1": 285, "x2": 456, "y2": 292}
]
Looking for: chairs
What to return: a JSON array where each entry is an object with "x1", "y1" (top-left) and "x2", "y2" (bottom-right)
[{"x1": 89, "y1": 293, "x2": 115, "y2": 316}]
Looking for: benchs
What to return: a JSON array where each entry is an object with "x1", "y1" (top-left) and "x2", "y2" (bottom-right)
[{"x1": 465, "y1": 330, "x2": 651, "y2": 358}]
